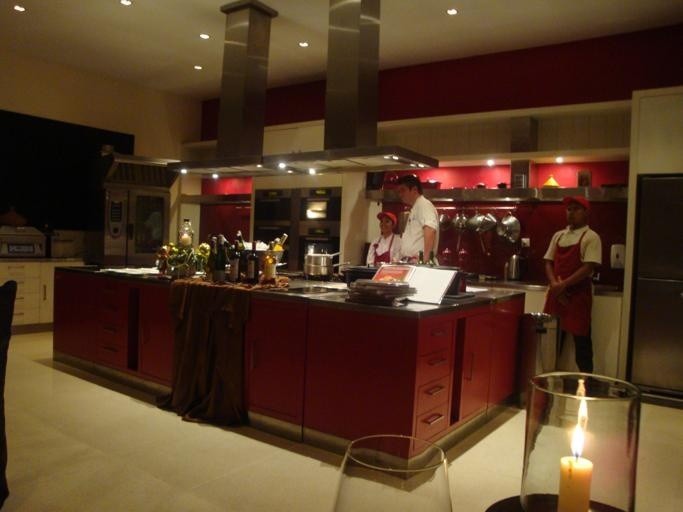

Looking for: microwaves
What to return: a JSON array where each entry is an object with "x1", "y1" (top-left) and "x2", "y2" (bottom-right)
[{"x1": 45, "y1": 224, "x2": 97, "y2": 259}]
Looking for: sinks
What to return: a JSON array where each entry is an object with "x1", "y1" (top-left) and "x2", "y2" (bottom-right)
[{"x1": 287, "y1": 284, "x2": 340, "y2": 296}]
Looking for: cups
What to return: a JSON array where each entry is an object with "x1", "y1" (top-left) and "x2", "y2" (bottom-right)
[
  {"x1": 331, "y1": 434, "x2": 452, "y2": 511},
  {"x1": 520, "y1": 372, "x2": 642, "y2": 512}
]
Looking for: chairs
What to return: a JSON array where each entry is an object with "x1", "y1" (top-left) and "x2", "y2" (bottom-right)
[{"x1": 0, "y1": 279, "x2": 17, "y2": 512}]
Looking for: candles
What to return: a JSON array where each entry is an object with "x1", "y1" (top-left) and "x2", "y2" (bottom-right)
[{"x1": 557, "y1": 426, "x2": 594, "y2": 512}]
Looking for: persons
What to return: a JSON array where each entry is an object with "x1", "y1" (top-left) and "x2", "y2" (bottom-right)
[
  {"x1": 363, "y1": 211, "x2": 405, "y2": 268},
  {"x1": 541, "y1": 195, "x2": 604, "y2": 396},
  {"x1": 400, "y1": 177, "x2": 439, "y2": 268}
]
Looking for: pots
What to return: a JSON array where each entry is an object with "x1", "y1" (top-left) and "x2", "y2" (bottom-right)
[
  {"x1": 302, "y1": 251, "x2": 352, "y2": 277},
  {"x1": 436, "y1": 210, "x2": 496, "y2": 234},
  {"x1": 495, "y1": 212, "x2": 521, "y2": 247}
]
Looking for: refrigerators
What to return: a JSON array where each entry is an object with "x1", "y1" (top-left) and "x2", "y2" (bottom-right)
[{"x1": 628, "y1": 176, "x2": 682, "y2": 396}]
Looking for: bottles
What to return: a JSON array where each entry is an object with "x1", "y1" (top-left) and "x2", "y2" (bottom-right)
[
  {"x1": 177, "y1": 218, "x2": 192, "y2": 249},
  {"x1": 229, "y1": 240, "x2": 241, "y2": 282},
  {"x1": 207, "y1": 241, "x2": 216, "y2": 281},
  {"x1": 263, "y1": 253, "x2": 276, "y2": 283},
  {"x1": 268, "y1": 233, "x2": 288, "y2": 250},
  {"x1": 245, "y1": 242, "x2": 259, "y2": 283},
  {"x1": 212, "y1": 244, "x2": 226, "y2": 283}
]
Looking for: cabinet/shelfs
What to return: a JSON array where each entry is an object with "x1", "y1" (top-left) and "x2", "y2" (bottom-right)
[
  {"x1": 448, "y1": 312, "x2": 488, "y2": 429},
  {"x1": 53, "y1": 268, "x2": 128, "y2": 369},
  {"x1": 263, "y1": 118, "x2": 326, "y2": 155},
  {"x1": 246, "y1": 294, "x2": 304, "y2": 424},
  {"x1": 305, "y1": 305, "x2": 458, "y2": 458},
  {"x1": 139, "y1": 281, "x2": 177, "y2": 387},
  {"x1": 0, "y1": 262, "x2": 40, "y2": 327},
  {"x1": 488, "y1": 298, "x2": 526, "y2": 407},
  {"x1": 41, "y1": 262, "x2": 83, "y2": 325},
  {"x1": 375, "y1": 98, "x2": 632, "y2": 164}
]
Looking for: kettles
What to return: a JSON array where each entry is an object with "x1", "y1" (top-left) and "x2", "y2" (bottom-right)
[{"x1": 506, "y1": 254, "x2": 523, "y2": 280}]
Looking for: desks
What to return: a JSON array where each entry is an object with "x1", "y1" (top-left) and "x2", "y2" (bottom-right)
[{"x1": 487, "y1": 491, "x2": 626, "y2": 512}]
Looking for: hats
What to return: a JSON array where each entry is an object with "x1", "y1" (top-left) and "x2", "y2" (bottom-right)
[
  {"x1": 563, "y1": 195, "x2": 590, "y2": 209},
  {"x1": 377, "y1": 211, "x2": 397, "y2": 225}
]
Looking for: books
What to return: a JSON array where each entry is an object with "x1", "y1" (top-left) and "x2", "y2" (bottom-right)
[
  {"x1": 370, "y1": 263, "x2": 457, "y2": 305},
  {"x1": 343, "y1": 279, "x2": 415, "y2": 306}
]
